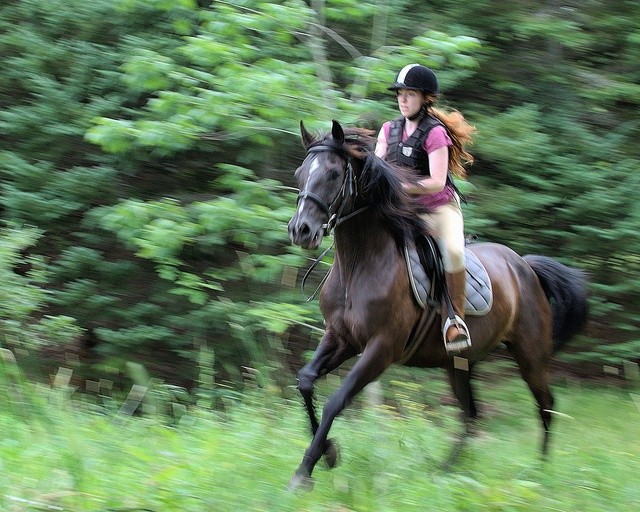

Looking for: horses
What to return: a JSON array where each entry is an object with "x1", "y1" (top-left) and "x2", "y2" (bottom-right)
[{"x1": 285, "y1": 118, "x2": 593, "y2": 496}]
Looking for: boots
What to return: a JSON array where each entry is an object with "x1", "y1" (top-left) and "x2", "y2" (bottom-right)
[{"x1": 441, "y1": 269, "x2": 468, "y2": 343}]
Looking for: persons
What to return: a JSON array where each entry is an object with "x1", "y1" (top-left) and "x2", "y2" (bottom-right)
[{"x1": 373, "y1": 63, "x2": 478, "y2": 352}]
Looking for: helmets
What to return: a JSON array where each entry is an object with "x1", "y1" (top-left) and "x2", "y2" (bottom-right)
[{"x1": 387, "y1": 63, "x2": 437, "y2": 93}]
